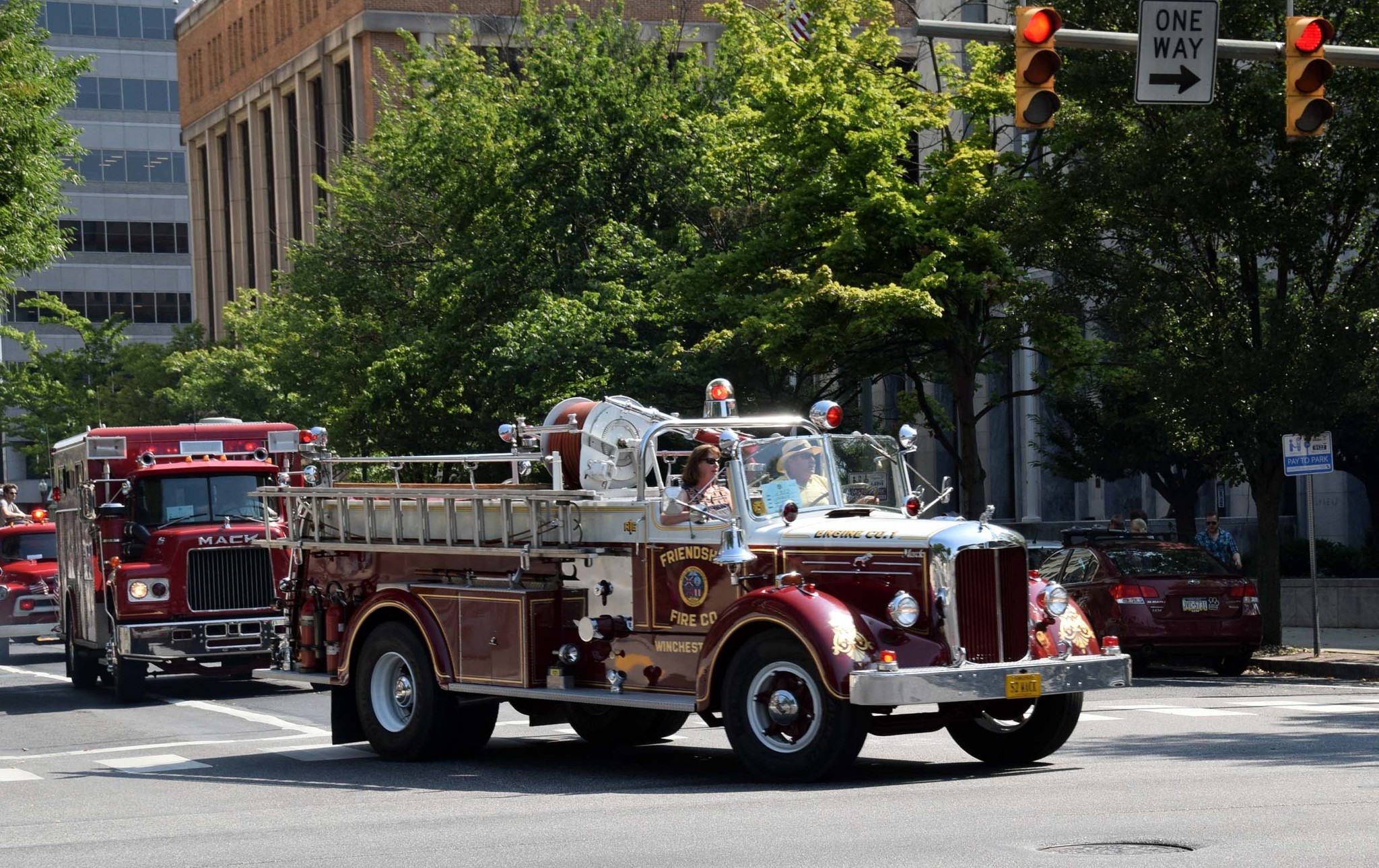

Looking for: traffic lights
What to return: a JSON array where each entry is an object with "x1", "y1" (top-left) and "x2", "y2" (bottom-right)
[
  {"x1": 1015, "y1": 3, "x2": 1063, "y2": 130},
  {"x1": 1285, "y1": 16, "x2": 1335, "y2": 136}
]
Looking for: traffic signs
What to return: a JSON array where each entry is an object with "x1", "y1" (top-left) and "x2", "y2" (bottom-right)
[{"x1": 1134, "y1": 0, "x2": 1221, "y2": 105}]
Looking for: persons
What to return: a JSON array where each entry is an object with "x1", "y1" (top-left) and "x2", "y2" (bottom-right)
[
  {"x1": 772, "y1": 440, "x2": 879, "y2": 507},
  {"x1": 0, "y1": 484, "x2": 32, "y2": 526},
  {"x1": 660, "y1": 445, "x2": 730, "y2": 525},
  {"x1": 221, "y1": 476, "x2": 276, "y2": 516},
  {"x1": 1195, "y1": 512, "x2": 1242, "y2": 569},
  {"x1": 1131, "y1": 510, "x2": 1148, "y2": 532},
  {"x1": 1110, "y1": 515, "x2": 1126, "y2": 530}
]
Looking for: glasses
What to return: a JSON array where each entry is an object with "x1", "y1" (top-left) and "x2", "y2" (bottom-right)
[
  {"x1": 8, "y1": 492, "x2": 17, "y2": 494},
  {"x1": 699, "y1": 458, "x2": 722, "y2": 464},
  {"x1": 1206, "y1": 521, "x2": 1216, "y2": 525},
  {"x1": 787, "y1": 452, "x2": 820, "y2": 461}
]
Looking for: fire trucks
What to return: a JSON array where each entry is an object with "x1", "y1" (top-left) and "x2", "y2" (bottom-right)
[{"x1": 48, "y1": 416, "x2": 342, "y2": 702}]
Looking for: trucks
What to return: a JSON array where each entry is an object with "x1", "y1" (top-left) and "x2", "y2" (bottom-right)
[
  {"x1": 0, "y1": 509, "x2": 63, "y2": 667},
  {"x1": 245, "y1": 376, "x2": 1133, "y2": 787}
]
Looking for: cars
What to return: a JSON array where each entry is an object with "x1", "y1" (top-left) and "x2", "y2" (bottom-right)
[{"x1": 1025, "y1": 524, "x2": 1265, "y2": 679}]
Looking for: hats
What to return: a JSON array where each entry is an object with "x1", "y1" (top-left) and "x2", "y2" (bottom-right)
[{"x1": 776, "y1": 440, "x2": 824, "y2": 473}]
